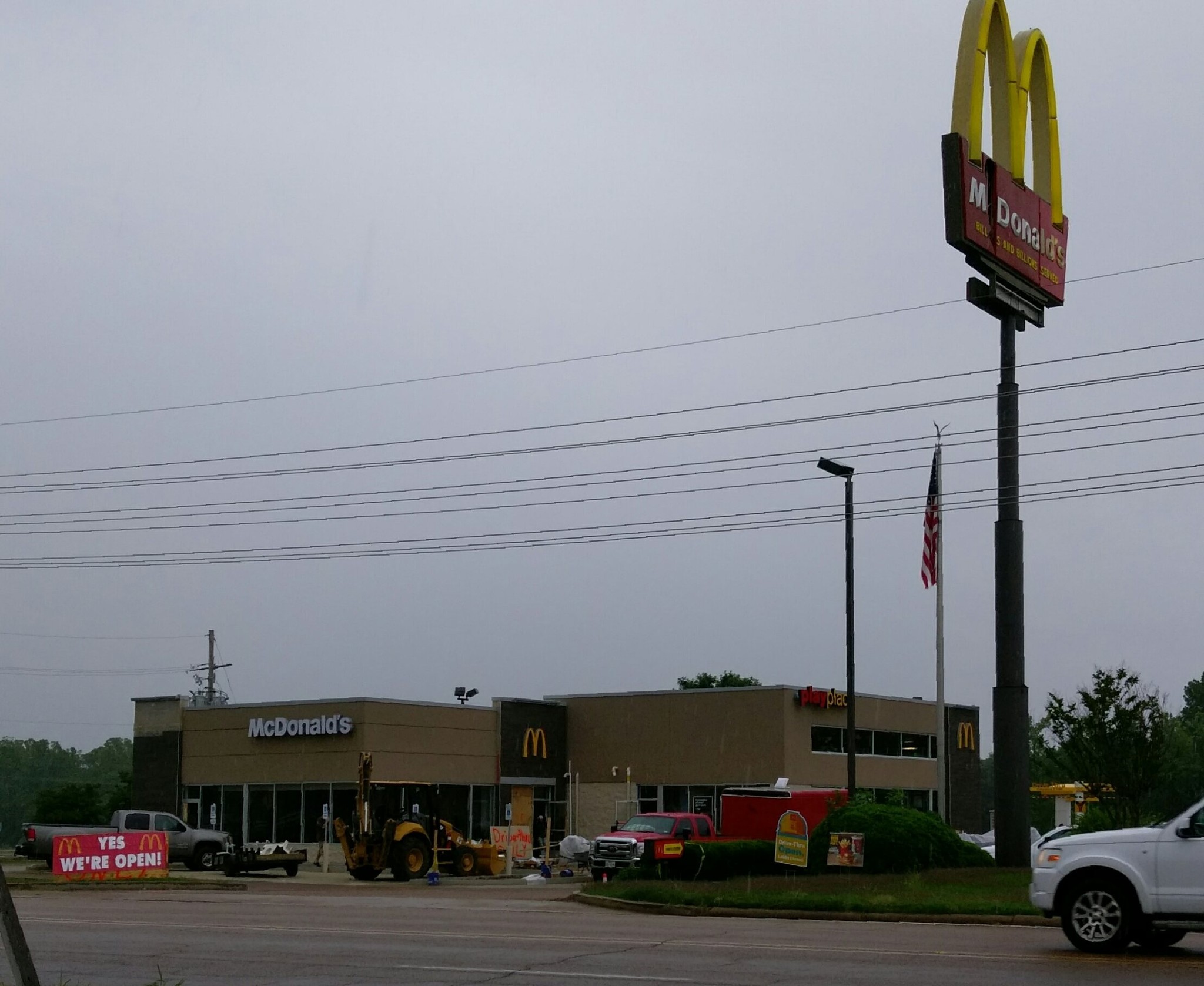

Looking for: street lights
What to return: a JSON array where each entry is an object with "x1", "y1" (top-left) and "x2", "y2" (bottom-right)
[{"x1": 816, "y1": 457, "x2": 860, "y2": 800}]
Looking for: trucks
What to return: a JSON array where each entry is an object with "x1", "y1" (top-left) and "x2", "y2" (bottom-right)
[{"x1": 720, "y1": 786, "x2": 848, "y2": 844}]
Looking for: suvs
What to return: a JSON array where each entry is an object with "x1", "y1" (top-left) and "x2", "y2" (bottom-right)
[
  {"x1": 587, "y1": 811, "x2": 752, "y2": 882},
  {"x1": 1027, "y1": 794, "x2": 1204, "y2": 954}
]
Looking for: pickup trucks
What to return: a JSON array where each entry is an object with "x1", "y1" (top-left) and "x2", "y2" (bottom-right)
[{"x1": 15, "y1": 808, "x2": 236, "y2": 872}]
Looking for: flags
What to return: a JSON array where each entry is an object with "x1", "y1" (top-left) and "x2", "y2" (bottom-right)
[{"x1": 920, "y1": 452, "x2": 938, "y2": 588}]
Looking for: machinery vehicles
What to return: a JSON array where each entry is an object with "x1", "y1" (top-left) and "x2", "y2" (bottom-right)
[{"x1": 329, "y1": 751, "x2": 509, "y2": 880}]
